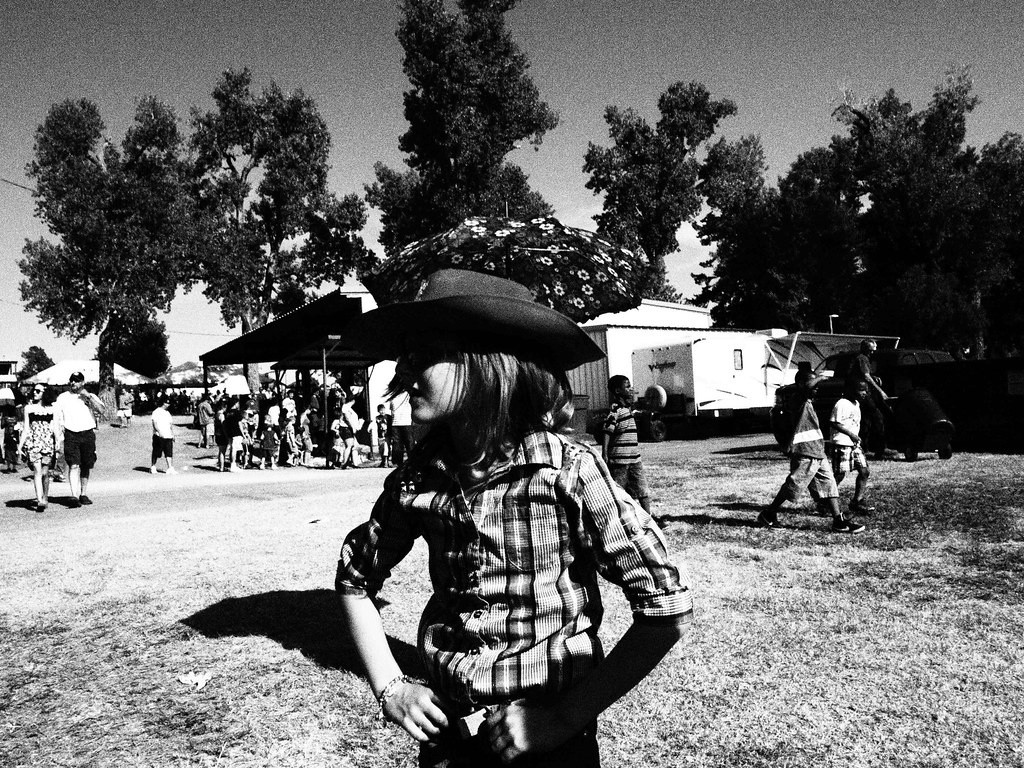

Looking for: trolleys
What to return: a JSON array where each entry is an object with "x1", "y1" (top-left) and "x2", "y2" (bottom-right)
[{"x1": 881, "y1": 396, "x2": 953, "y2": 461}]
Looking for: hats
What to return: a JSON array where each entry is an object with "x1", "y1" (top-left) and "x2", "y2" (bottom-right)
[
  {"x1": 793, "y1": 370, "x2": 823, "y2": 391},
  {"x1": 346, "y1": 268, "x2": 609, "y2": 373},
  {"x1": 69, "y1": 372, "x2": 84, "y2": 381}
]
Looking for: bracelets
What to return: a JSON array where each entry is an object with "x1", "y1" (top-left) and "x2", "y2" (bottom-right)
[{"x1": 375, "y1": 675, "x2": 428, "y2": 721}]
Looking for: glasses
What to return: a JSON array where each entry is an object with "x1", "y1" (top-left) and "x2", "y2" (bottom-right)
[
  {"x1": 33, "y1": 389, "x2": 46, "y2": 394},
  {"x1": 70, "y1": 379, "x2": 82, "y2": 383}
]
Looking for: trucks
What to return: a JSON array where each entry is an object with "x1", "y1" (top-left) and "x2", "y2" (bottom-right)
[{"x1": 769, "y1": 347, "x2": 1024, "y2": 462}]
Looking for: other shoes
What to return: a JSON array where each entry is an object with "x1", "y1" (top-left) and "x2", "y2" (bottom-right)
[
  {"x1": 850, "y1": 498, "x2": 875, "y2": 512},
  {"x1": 79, "y1": 495, "x2": 92, "y2": 504},
  {"x1": 150, "y1": 465, "x2": 157, "y2": 475},
  {"x1": 167, "y1": 468, "x2": 176, "y2": 475},
  {"x1": 69, "y1": 497, "x2": 81, "y2": 508},
  {"x1": 38, "y1": 495, "x2": 48, "y2": 512},
  {"x1": 814, "y1": 507, "x2": 835, "y2": 517}
]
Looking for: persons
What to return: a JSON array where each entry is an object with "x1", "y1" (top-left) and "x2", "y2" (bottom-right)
[
  {"x1": 119, "y1": 388, "x2": 134, "y2": 428},
  {"x1": 332, "y1": 268, "x2": 694, "y2": 768},
  {"x1": 130, "y1": 387, "x2": 198, "y2": 414},
  {"x1": 54, "y1": 372, "x2": 106, "y2": 507},
  {"x1": 815, "y1": 370, "x2": 875, "y2": 514},
  {"x1": 757, "y1": 370, "x2": 866, "y2": 534},
  {"x1": 150, "y1": 398, "x2": 178, "y2": 476},
  {"x1": 17, "y1": 383, "x2": 64, "y2": 509},
  {"x1": 602, "y1": 375, "x2": 649, "y2": 514},
  {"x1": 196, "y1": 378, "x2": 416, "y2": 472},
  {"x1": 847, "y1": 337, "x2": 898, "y2": 456}
]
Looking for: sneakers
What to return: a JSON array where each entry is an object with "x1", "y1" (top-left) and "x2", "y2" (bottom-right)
[
  {"x1": 757, "y1": 507, "x2": 782, "y2": 529},
  {"x1": 831, "y1": 513, "x2": 865, "y2": 534}
]
